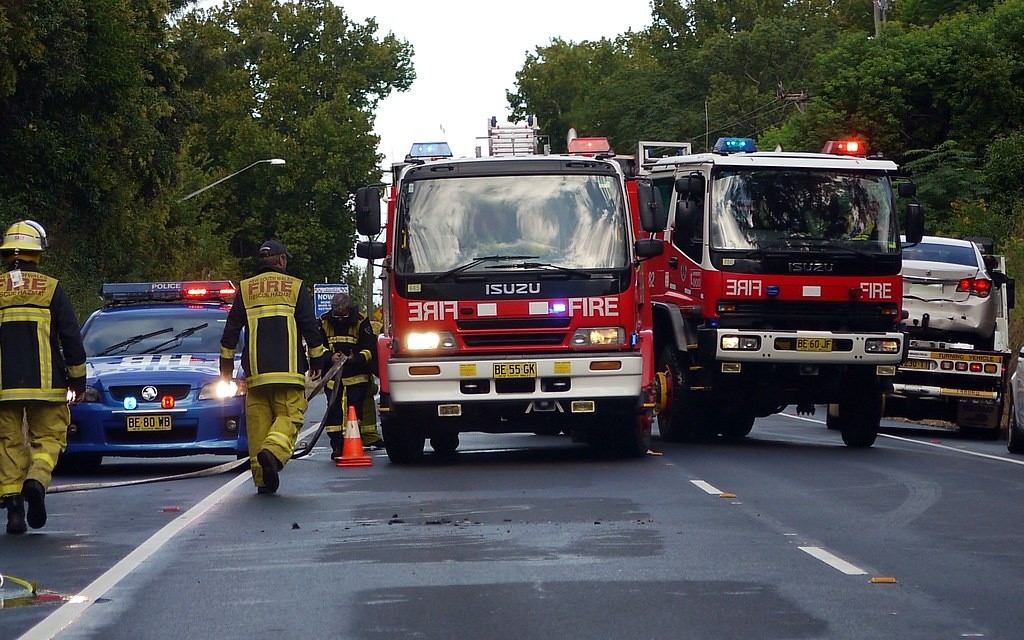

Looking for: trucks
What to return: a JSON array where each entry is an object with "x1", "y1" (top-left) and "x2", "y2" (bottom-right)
[{"x1": 827, "y1": 236, "x2": 1015, "y2": 442}]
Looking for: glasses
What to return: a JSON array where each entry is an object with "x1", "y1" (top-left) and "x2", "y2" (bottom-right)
[{"x1": 331, "y1": 313, "x2": 349, "y2": 320}]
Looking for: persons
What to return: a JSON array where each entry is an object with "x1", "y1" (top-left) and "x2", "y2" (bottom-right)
[
  {"x1": 0, "y1": 221, "x2": 87, "y2": 534},
  {"x1": 320, "y1": 294, "x2": 385, "y2": 460},
  {"x1": 760, "y1": 170, "x2": 879, "y2": 241},
  {"x1": 220, "y1": 240, "x2": 323, "y2": 493}
]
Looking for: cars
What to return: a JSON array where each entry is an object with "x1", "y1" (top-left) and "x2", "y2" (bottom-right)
[
  {"x1": 1007, "y1": 343, "x2": 1024, "y2": 454},
  {"x1": 47, "y1": 280, "x2": 251, "y2": 479},
  {"x1": 892, "y1": 237, "x2": 999, "y2": 351}
]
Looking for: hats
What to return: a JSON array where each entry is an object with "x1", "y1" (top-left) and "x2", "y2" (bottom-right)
[{"x1": 261, "y1": 240, "x2": 294, "y2": 260}]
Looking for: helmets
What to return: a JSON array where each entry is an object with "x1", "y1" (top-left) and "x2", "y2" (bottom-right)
[{"x1": 2, "y1": 219, "x2": 49, "y2": 254}]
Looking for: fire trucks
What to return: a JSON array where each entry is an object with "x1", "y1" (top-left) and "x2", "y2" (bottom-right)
[
  {"x1": 355, "y1": 115, "x2": 665, "y2": 466},
  {"x1": 630, "y1": 137, "x2": 925, "y2": 450}
]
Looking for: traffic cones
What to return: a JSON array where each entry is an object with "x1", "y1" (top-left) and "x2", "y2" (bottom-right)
[{"x1": 333, "y1": 405, "x2": 374, "y2": 468}]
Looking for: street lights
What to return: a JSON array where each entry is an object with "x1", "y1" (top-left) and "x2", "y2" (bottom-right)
[{"x1": 178, "y1": 159, "x2": 286, "y2": 201}]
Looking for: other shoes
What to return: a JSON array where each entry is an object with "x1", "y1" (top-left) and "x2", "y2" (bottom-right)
[
  {"x1": 366, "y1": 440, "x2": 386, "y2": 449},
  {"x1": 331, "y1": 448, "x2": 344, "y2": 461},
  {"x1": 24, "y1": 479, "x2": 47, "y2": 529},
  {"x1": 256, "y1": 487, "x2": 271, "y2": 494},
  {"x1": 6, "y1": 495, "x2": 28, "y2": 535},
  {"x1": 256, "y1": 449, "x2": 281, "y2": 492}
]
